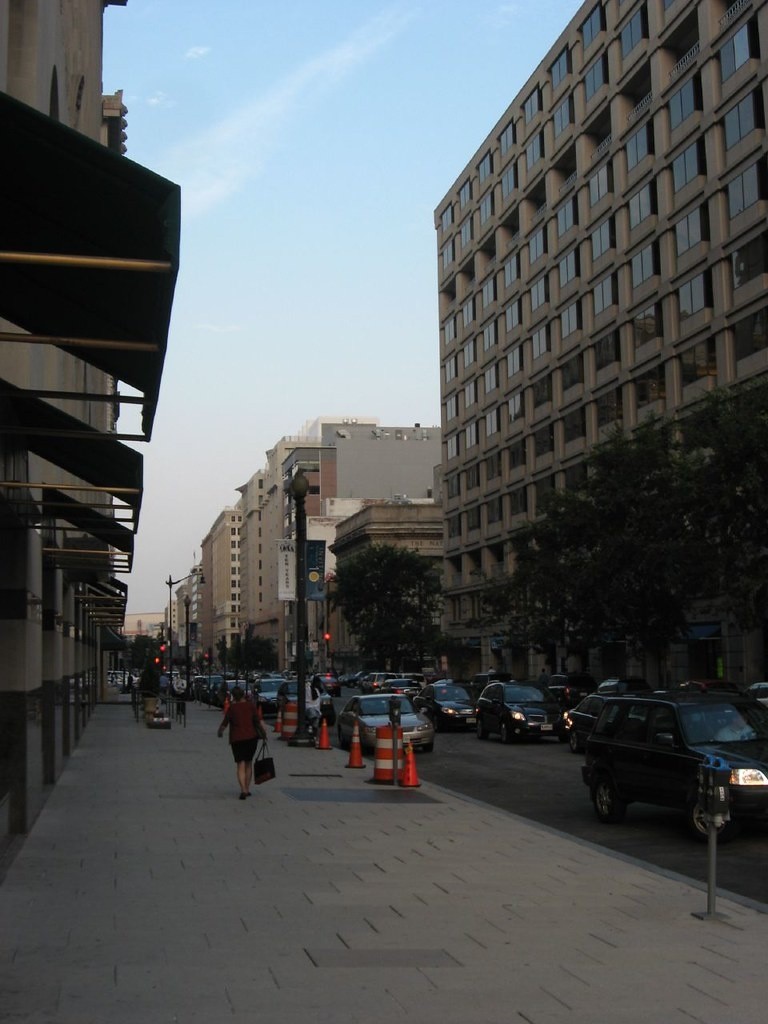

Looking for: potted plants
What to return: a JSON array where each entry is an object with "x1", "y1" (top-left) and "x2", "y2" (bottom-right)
[{"x1": 139, "y1": 658, "x2": 161, "y2": 713}]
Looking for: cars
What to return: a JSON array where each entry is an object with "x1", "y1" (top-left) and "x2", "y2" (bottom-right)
[
  {"x1": 338, "y1": 694, "x2": 434, "y2": 757},
  {"x1": 109, "y1": 671, "x2": 513, "y2": 732}
]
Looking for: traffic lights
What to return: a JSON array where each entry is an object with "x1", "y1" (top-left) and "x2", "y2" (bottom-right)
[{"x1": 325, "y1": 635, "x2": 330, "y2": 641}]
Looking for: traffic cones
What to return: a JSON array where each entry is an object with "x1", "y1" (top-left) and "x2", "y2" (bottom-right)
[
  {"x1": 274, "y1": 707, "x2": 283, "y2": 732},
  {"x1": 257, "y1": 705, "x2": 267, "y2": 730},
  {"x1": 401, "y1": 744, "x2": 421, "y2": 786},
  {"x1": 223, "y1": 699, "x2": 229, "y2": 715},
  {"x1": 345, "y1": 720, "x2": 366, "y2": 768},
  {"x1": 315, "y1": 718, "x2": 333, "y2": 749}
]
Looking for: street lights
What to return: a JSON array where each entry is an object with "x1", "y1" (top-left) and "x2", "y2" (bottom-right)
[
  {"x1": 160, "y1": 623, "x2": 165, "y2": 643},
  {"x1": 165, "y1": 573, "x2": 207, "y2": 697},
  {"x1": 183, "y1": 595, "x2": 191, "y2": 701},
  {"x1": 290, "y1": 468, "x2": 316, "y2": 747}
]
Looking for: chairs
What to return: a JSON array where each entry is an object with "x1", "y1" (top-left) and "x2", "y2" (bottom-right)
[{"x1": 624, "y1": 716, "x2": 730, "y2": 747}]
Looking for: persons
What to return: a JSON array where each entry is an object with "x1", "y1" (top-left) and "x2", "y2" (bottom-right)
[
  {"x1": 110, "y1": 670, "x2": 118, "y2": 688},
  {"x1": 539, "y1": 668, "x2": 548, "y2": 684},
  {"x1": 489, "y1": 665, "x2": 495, "y2": 672},
  {"x1": 173, "y1": 675, "x2": 187, "y2": 715},
  {"x1": 218, "y1": 687, "x2": 268, "y2": 799},
  {"x1": 306, "y1": 677, "x2": 321, "y2": 745},
  {"x1": 126, "y1": 672, "x2": 133, "y2": 693}
]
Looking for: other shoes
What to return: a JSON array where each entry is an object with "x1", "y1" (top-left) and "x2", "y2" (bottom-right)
[{"x1": 239, "y1": 792, "x2": 252, "y2": 800}]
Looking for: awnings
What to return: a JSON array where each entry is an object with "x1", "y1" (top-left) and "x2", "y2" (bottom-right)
[
  {"x1": 103, "y1": 627, "x2": 127, "y2": 649},
  {"x1": 74, "y1": 575, "x2": 128, "y2": 628},
  {"x1": 42, "y1": 483, "x2": 135, "y2": 576},
  {"x1": 0, "y1": 93, "x2": 180, "y2": 442},
  {"x1": 0, "y1": 378, "x2": 143, "y2": 534}
]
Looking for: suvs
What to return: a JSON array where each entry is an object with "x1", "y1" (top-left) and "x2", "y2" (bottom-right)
[
  {"x1": 545, "y1": 673, "x2": 768, "y2": 754},
  {"x1": 581, "y1": 694, "x2": 768, "y2": 842},
  {"x1": 475, "y1": 682, "x2": 568, "y2": 744}
]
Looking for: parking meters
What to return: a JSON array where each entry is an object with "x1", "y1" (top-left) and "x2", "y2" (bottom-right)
[
  {"x1": 696, "y1": 754, "x2": 731, "y2": 920},
  {"x1": 389, "y1": 695, "x2": 401, "y2": 787}
]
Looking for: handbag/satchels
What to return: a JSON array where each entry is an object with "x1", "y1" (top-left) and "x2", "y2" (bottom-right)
[{"x1": 254, "y1": 741, "x2": 275, "y2": 783}]
